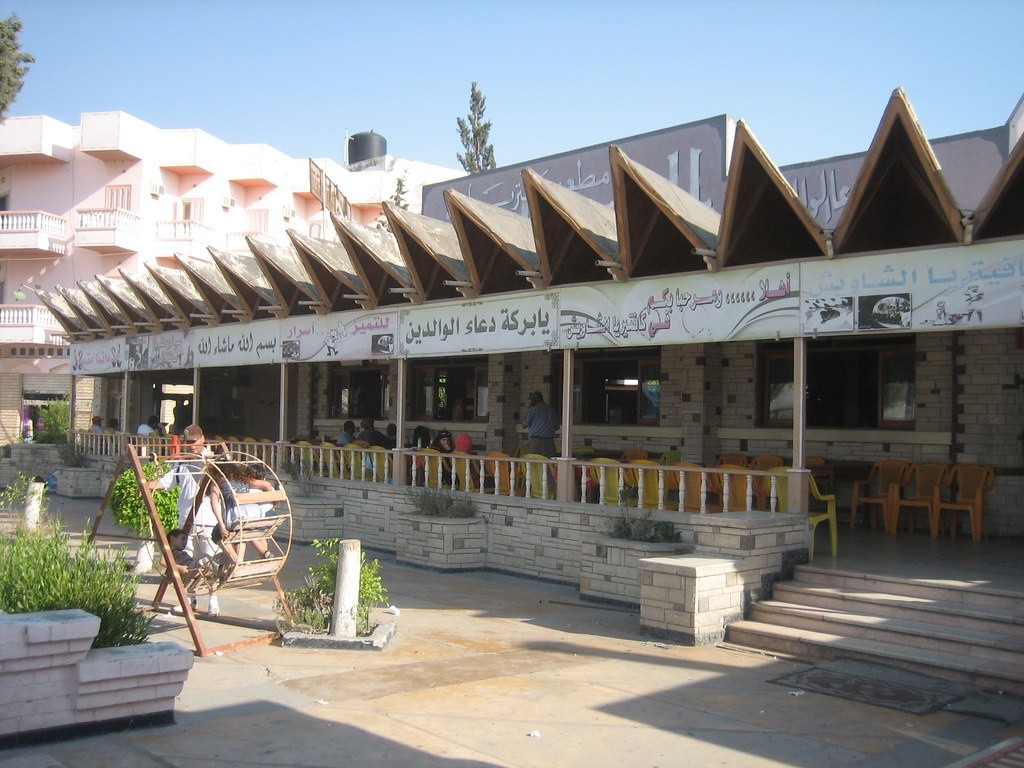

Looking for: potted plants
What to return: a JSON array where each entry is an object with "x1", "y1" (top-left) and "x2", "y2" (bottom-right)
[
  {"x1": 395, "y1": 487, "x2": 490, "y2": 575},
  {"x1": 579, "y1": 487, "x2": 696, "y2": 612},
  {"x1": 269, "y1": 454, "x2": 344, "y2": 546}
]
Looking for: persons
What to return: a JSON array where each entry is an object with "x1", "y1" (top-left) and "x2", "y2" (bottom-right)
[
  {"x1": 90, "y1": 416, "x2": 104, "y2": 439},
  {"x1": 339, "y1": 420, "x2": 356, "y2": 445},
  {"x1": 137, "y1": 416, "x2": 162, "y2": 438},
  {"x1": 357, "y1": 417, "x2": 396, "y2": 450},
  {"x1": 146, "y1": 425, "x2": 222, "y2": 618},
  {"x1": 432, "y1": 430, "x2": 453, "y2": 486},
  {"x1": 520, "y1": 391, "x2": 560, "y2": 457},
  {"x1": 103, "y1": 418, "x2": 120, "y2": 446},
  {"x1": 454, "y1": 434, "x2": 494, "y2": 489},
  {"x1": 159, "y1": 529, "x2": 227, "y2": 584},
  {"x1": 208, "y1": 460, "x2": 279, "y2": 561}
]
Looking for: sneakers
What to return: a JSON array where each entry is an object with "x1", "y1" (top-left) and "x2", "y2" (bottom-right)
[
  {"x1": 208, "y1": 603, "x2": 220, "y2": 616},
  {"x1": 172, "y1": 603, "x2": 196, "y2": 613}
]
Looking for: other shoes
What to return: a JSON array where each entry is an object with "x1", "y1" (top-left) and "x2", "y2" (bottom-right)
[{"x1": 264, "y1": 551, "x2": 273, "y2": 559}]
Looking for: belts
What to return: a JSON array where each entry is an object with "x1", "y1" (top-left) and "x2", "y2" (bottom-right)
[{"x1": 530, "y1": 436, "x2": 554, "y2": 439}]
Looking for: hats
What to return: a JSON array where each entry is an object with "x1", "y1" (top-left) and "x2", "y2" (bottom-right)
[
  {"x1": 184, "y1": 425, "x2": 202, "y2": 441},
  {"x1": 93, "y1": 416, "x2": 103, "y2": 422},
  {"x1": 530, "y1": 391, "x2": 543, "y2": 405},
  {"x1": 361, "y1": 417, "x2": 373, "y2": 427}
]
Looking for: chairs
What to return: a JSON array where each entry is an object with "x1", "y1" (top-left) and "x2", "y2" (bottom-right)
[
  {"x1": 936, "y1": 464, "x2": 996, "y2": 543},
  {"x1": 891, "y1": 462, "x2": 949, "y2": 535},
  {"x1": 78, "y1": 428, "x2": 837, "y2": 563},
  {"x1": 850, "y1": 458, "x2": 904, "y2": 527}
]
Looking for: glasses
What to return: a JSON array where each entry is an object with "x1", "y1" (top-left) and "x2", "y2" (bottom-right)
[{"x1": 439, "y1": 433, "x2": 450, "y2": 437}]
